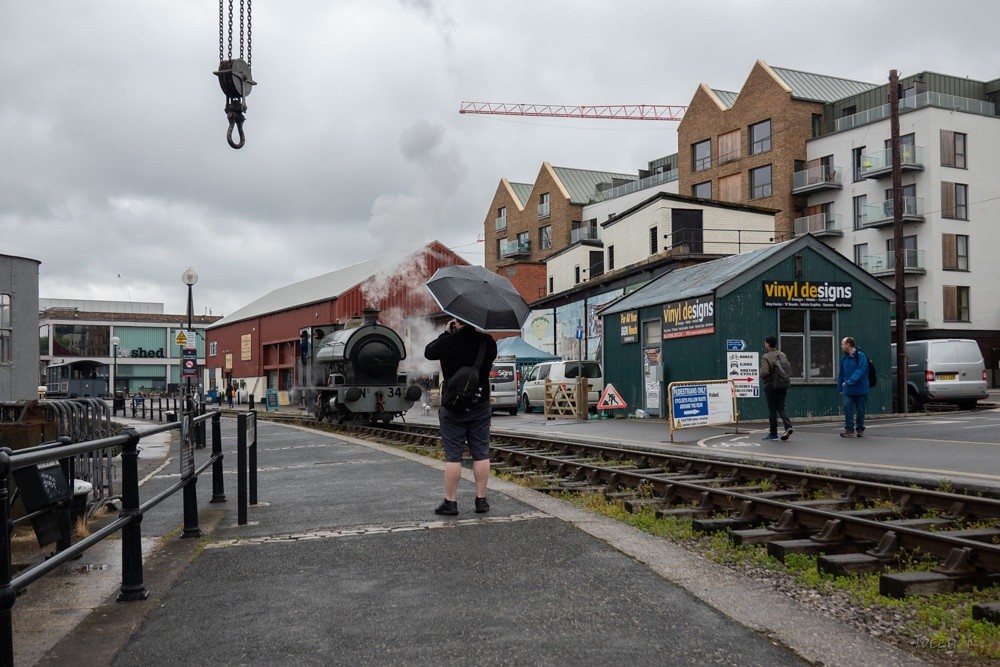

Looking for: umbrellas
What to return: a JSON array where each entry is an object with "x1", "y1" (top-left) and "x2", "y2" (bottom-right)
[{"x1": 425, "y1": 265, "x2": 533, "y2": 333}]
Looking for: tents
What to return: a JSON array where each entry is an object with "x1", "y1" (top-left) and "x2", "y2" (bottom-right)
[{"x1": 494, "y1": 337, "x2": 563, "y2": 412}]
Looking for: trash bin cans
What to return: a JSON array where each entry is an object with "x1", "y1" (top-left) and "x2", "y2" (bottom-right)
[
  {"x1": 133, "y1": 398, "x2": 145, "y2": 418},
  {"x1": 113, "y1": 397, "x2": 126, "y2": 417},
  {"x1": 207, "y1": 390, "x2": 217, "y2": 402}
]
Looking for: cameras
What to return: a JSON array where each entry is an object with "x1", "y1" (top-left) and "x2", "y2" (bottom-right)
[{"x1": 450, "y1": 322, "x2": 459, "y2": 334}]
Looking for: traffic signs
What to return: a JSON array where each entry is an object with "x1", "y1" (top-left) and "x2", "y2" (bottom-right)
[{"x1": 727, "y1": 339, "x2": 746, "y2": 351}]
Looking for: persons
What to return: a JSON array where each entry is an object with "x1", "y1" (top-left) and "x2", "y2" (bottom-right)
[
  {"x1": 425, "y1": 318, "x2": 498, "y2": 514},
  {"x1": 760, "y1": 336, "x2": 794, "y2": 440},
  {"x1": 226, "y1": 382, "x2": 234, "y2": 408},
  {"x1": 836, "y1": 337, "x2": 870, "y2": 437}
]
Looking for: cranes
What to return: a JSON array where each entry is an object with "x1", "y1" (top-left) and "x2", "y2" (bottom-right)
[{"x1": 459, "y1": 101, "x2": 688, "y2": 121}]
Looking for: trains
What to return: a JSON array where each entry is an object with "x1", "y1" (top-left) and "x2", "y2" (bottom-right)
[{"x1": 289, "y1": 308, "x2": 422, "y2": 427}]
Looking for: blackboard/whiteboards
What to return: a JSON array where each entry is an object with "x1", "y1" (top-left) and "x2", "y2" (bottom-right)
[{"x1": 266, "y1": 388, "x2": 279, "y2": 409}]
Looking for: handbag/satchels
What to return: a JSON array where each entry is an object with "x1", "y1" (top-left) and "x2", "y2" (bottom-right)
[{"x1": 442, "y1": 367, "x2": 490, "y2": 407}]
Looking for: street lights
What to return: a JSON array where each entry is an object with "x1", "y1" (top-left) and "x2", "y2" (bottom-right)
[
  {"x1": 111, "y1": 336, "x2": 121, "y2": 416},
  {"x1": 182, "y1": 268, "x2": 198, "y2": 412}
]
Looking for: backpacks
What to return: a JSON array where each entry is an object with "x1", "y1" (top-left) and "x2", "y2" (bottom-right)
[
  {"x1": 767, "y1": 350, "x2": 792, "y2": 389},
  {"x1": 854, "y1": 348, "x2": 877, "y2": 388}
]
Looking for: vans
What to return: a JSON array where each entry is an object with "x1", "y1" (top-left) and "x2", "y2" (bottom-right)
[
  {"x1": 891, "y1": 339, "x2": 989, "y2": 413},
  {"x1": 488, "y1": 363, "x2": 520, "y2": 415},
  {"x1": 522, "y1": 360, "x2": 604, "y2": 414}
]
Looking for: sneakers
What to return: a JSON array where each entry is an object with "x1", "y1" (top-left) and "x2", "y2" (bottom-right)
[
  {"x1": 840, "y1": 430, "x2": 855, "y2": 436},
  {"x1": 762, "y1": 434, "x2": 779, "y2": 441},
  {"x1": 475, "y1": 496, "x2": 490, "y2": 512},
  {"x1": 781, "y1": 428, "x2": 793, "y2": 440},
  {"x1": 435, "y1": 499, "x2": 459, "y2": 515},
  {"x1": 857, "y1": 431, "x2": 863, "y2": 436}
]
